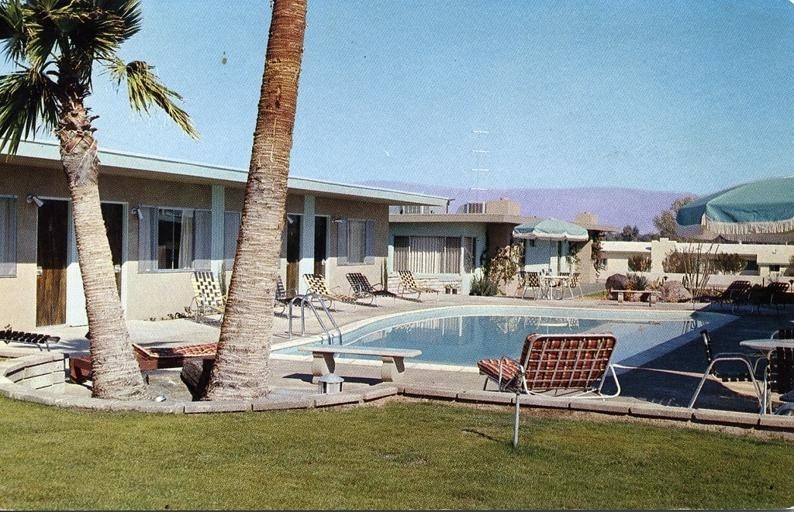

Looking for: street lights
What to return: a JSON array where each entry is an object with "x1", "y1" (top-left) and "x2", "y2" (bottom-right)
[{"x1": 4, "y1": 324, "x2": 12, "y2": 344}]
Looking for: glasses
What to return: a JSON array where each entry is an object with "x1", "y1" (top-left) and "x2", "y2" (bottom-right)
[
  {"x1": 130, "y1": 206, "x2": 143, "y2": 221},
  {"x1": 286, "y1": 215, "x2": 295, "y2": 224},
  {"x1": 332, "y1": 215, "x2": 344, "y2": 224},
  {"x1": 26, "y1": 196, "x2": 43, "y2": 207}
]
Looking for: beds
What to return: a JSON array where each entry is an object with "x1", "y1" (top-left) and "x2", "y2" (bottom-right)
[
  {"x1": 513, "y1": 217, "x2": 589, "y2": 300},
  {"x1": 675, "y1": 175, "x2": 793, "y2": 241}
]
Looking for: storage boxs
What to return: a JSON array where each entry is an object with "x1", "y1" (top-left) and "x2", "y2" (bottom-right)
[
  {"x1": 299, "y1": 345, "x2": 422, "y2": 384},
  {"x1": 611, "y1": 290, "x2": 662, "y2": 307}
]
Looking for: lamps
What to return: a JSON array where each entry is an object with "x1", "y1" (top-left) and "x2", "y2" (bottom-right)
[
  {"x1": 688, "y1": 280, "x2": 794, "y2": 417},
  {"x1": 273, "y1": 270, "x2": 440, "y2": 316},
  {"x1": 189, "y1": 270, "x2": 226, "y2": 324},
  {"x1": 69, "y1": 331, "x2": 218, "y2": 383},
  {"x1": 480, "y1": 331, "x2": 622, "y2": 398},
  {"x1": 513, "y1": 270, "x2": 584, "y2": 300}
]
